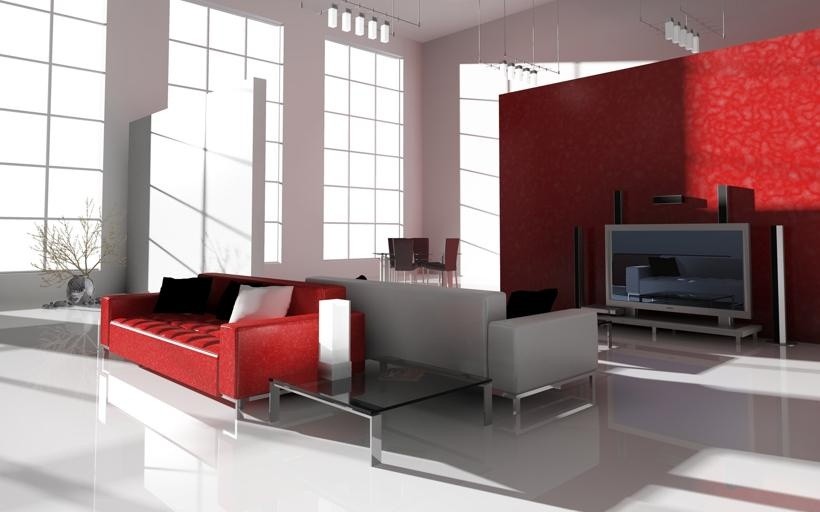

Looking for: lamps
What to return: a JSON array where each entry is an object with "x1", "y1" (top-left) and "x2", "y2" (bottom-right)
[{"x1": 315, "y1": 298, "x2": 353, "y2": 382}]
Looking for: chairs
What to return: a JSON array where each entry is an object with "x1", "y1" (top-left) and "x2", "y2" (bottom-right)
[{"x1": 388, "y1": 237, "x2": 460, "y2": 288}]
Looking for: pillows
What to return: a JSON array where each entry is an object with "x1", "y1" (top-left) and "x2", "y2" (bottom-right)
[
  {"x1": 506, "y1": 288, "x2": 558, "y2": 321},
  {"x1": 152, "y1": 276, "x2": 295, "y2": 324}
]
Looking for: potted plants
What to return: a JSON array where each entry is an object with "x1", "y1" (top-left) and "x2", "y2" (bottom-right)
[{"x1": 24, "y1": 194, "x2": 128, "y2": 302}]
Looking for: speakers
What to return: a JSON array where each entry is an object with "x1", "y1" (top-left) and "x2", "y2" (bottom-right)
[
  {"x1": 612, "y1": 190, "x2": 623, "y2": 224},
  {"x1": 717, "y1": 185, "x2": 729, "y2": 224},
  {"x1": 771, "y1": 225, "x2": 787, "y2": 345},
  {"x1": 572, "y1": 227, "x2": 582, "y2": 308},
  {"x1": 651, "y1": 195, "x2": 684, "y2": 204}
]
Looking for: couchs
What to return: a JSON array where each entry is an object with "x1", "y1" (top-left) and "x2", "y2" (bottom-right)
[
  {"x1": 304, "y1": 275, "x2": 600, "y2": 416},
  {"x1": 99, "y1": 272, "x2": 367, "y2": 421}
]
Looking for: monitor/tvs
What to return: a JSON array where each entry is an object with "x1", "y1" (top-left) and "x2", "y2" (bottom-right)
[{"x1": 604, "y1": 222, "x2": 754, "y2": 319}]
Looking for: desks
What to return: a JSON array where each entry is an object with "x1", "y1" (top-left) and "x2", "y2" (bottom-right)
[
  {"x1": 371, "y1": 250, "x2": 462, "y2": 282},
  {"x1": 598, "y1": 312, "x2": 763, "y2": 345}
]
